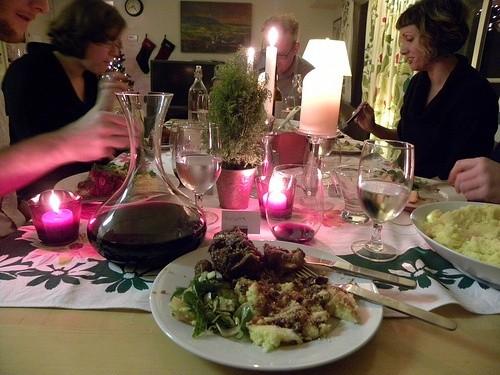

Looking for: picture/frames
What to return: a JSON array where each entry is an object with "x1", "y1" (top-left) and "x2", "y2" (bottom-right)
[{"x1": 333, "y1": 17, "x2": 342, "y2": 39}]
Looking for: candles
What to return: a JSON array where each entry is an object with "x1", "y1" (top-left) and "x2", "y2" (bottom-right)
[
  {"x1": 42, "y1": 189, "x2": 74, "y2": 241},
  {"x1": 262, "y1": 27, "x2": 278, "y2": 130},
  {"x1": 262, "y1": 176, "x2": 287, "y2": 211},
  {"x1": 299, "y1": 39, "x2": 346, "y2": 136},
  {"x1": 247, "y1": 48, "x2": 254, "y2": 79}
]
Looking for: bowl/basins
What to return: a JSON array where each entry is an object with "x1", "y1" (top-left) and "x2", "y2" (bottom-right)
[{"x1": 410, "y1": 200, "x2": 500, "y2": 290}]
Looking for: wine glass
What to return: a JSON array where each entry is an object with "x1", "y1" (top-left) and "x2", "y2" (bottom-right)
[
  {"x1": 352, "y1": 138, "x2": 416, "y2": 263},
  {"x1": 171, "y1": 121, "x2": 223, "y2": 226}
]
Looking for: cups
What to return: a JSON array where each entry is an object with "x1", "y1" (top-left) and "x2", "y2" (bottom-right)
[
  {"x1": 197, "y1": 94, "x2": 208, "y2": 122},
  {"x1": 255, "y1": 133, "x2": 389, "y2": 244},
  {"x1": 27, "y1": 189, "x2": 83, "y2": 247}
]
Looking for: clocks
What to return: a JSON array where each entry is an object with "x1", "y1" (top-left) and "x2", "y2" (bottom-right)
[{"x1": 125, "y1": 0, "x2": 143, "y2": 17}]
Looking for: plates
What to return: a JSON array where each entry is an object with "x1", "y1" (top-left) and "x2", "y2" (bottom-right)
[
  {"x1": 53, "y1": 170, "x2": 180, "y2": 204},
  {"x1": 143, "y1": 137, "x2": 191, "y2": 149},
  {"x1": 150, "y1": 239, "x2": 383, "y2": 371},
  {"x1": 273, "y1": 119, "x2": 299, "y2": 132},
  {"x1": 402, "y1": 175, "x2": 468, "y2": 213},
  {"x1": 331, "y1": 138, "x2": 363, "y2": 153}
]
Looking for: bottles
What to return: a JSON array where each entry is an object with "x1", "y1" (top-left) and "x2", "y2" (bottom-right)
[
  {"x1": 188, "y1": 66, "x2": 208, "y2": 121},
  {"x1": 87, "y1": 90, "x2": 208, "y2": 270}
]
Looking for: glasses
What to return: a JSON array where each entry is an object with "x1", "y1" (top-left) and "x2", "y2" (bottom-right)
[{"x1": 260, "y1": 39, "x2": 295, "y2": 59}]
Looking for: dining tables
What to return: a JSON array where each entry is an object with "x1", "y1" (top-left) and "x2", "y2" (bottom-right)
[{"x1": 0, "y1": 120, "x2": 500, "y2": 375}]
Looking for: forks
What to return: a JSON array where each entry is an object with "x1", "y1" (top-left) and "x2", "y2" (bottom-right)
[
  {"x1": 295, "y1": 265, "x2": 457, "y2": 331},
  {"x1": 337, "y1": 100, "x2": 367, "y2": 133}
]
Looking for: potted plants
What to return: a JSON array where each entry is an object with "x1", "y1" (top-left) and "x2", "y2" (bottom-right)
[{"x1": 199, "y1": 52, "x2": 275, "y2": 210}]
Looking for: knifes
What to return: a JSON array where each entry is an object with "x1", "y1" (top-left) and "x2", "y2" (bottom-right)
[{"x1": 304, "y1": 255, "x2": 417, "y2": 289}]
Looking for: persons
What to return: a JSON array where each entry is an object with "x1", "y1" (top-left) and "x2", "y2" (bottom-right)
[
  {"x1": 0, "y1": 0, "x2": 143, "y2": 237},
  {"x1": 352, "y1": 0, "x2": 500, "y2": 204},
  {"x1": 212, "y1": 15, "x2": 314, "y2": 122}
]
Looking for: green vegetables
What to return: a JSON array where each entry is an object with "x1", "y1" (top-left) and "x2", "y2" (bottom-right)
[
  {"x1": 375, "y1": 168, "x2": 426, "y2": 191},
  {"x1": 79, "y1": 163, "x2": 155, "y2": 185},
  {"x1": 171, "y1": 270, "x2": 252, "y2": 343}
]
